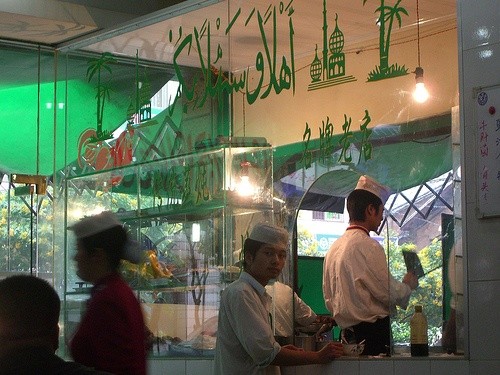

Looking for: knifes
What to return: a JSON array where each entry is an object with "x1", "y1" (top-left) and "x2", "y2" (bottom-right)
[{"x1": 402, "y1": 250, "x2": 425, "y2": 277}]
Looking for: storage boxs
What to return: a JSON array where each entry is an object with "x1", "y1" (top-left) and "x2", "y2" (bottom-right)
[
  {"x1": 140, "y1": 303, "x2": 219, "y2": 348},
  {"x1": 158, "y1": 270, "x2": 220, "y2": 304}
]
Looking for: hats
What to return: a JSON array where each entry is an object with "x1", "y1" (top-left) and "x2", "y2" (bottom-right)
[
  {"x1": 353, "y1": 175, "x2": 392, "y2": 206},
  {"x1": 248, "y1": 223, "x2": 289, "y2": 247},
  {"x1": 73, "y1": 210, "x2": 119, "y2": 238}
]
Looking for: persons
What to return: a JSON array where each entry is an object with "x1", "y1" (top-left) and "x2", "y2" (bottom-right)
[
  {"x1": 69, "y1": 210, "x2": 150, "y2": 375},
  {"x1": 264, "y1": 277, "x2": 317, "y2": 346},
  {"x1": 323, "y1": 176, "x2": 419, "y2": 355},
  {"x1": 0, "y1": 275, "x2": 116, "y2": 375},
  {"x1": 213, "y1": 223, "x2": 345, "y2": 375}
]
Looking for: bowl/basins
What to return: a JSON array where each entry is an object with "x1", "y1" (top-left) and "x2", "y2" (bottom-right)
[
  {"x1": 298, "y1": 323, "x2": 333, "y2": 333},
  {"x1": 315, "y1": 341, "x2": 328, "y2": 351},
  {"x1": 294, "y1": 335, "x2": 317, "y2": 351},
  {"x1": 343, "y1": 344, "x2": 364, "y2": 356}
]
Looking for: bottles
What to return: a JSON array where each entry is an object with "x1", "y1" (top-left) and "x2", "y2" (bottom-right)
[{"x1": 410, "y1": 305, "x2": 429, "y2": 357}]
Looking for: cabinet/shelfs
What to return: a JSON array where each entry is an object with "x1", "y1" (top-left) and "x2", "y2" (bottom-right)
[{"x1": 64, "y1": 146, "x2": 273, "y2": 359}]
